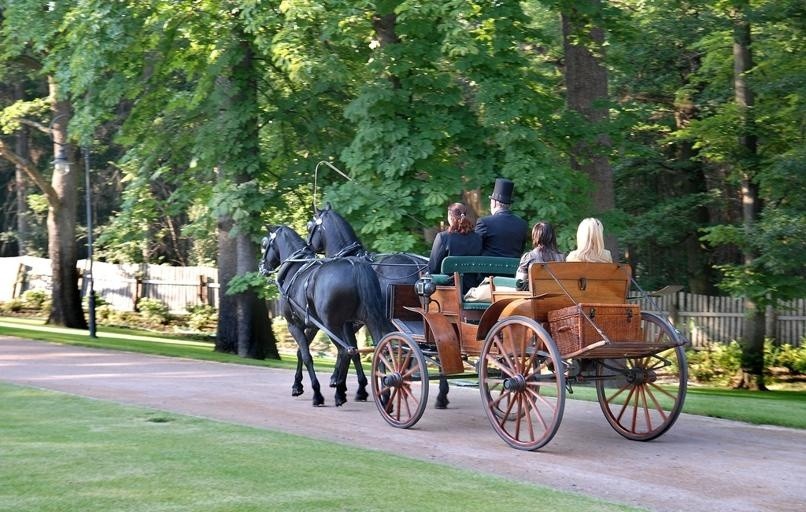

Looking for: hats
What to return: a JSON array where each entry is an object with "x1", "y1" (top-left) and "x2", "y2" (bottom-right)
[{"x1": 488, "y1": 177, "x2": 514, "y2": 204}]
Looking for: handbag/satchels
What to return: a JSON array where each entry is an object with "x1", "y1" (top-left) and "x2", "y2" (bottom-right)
[{"x1": 464, "y1": 277, "x2": 493, "y2": 303}]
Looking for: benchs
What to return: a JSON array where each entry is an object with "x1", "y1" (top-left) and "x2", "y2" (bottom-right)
[
  {"x1": 454, "y1": 271, "x2": 516, "y2": 356},
  {"x1": 501, "y1": 263, "x2": 632, "y2": 354},
  {"x1": 417, "y1": 255, "x2": 522, "y2": 344}
]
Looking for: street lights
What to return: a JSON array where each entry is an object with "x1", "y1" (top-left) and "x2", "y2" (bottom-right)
[{"x1": 48, "y1": 114, "x2": 97, "y2": 338}]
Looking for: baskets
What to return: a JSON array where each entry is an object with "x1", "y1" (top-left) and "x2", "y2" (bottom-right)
[{"x1": 551, "y1": 315, "x2": 642, "y2": 355}]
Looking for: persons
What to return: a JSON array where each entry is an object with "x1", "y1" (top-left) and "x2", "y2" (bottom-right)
[
  {"x1": 566, "y1": 216, "x2": 613, "y2": 263},
  {"x1": 425, "y1": 203, "x2": 483, "y2": 294},
  {"x1": 472, "y1": 179, "x2": 529, "y2": 289},
  {"x1": 514, "y1": 221, "x2": 566, "y2": 291}
]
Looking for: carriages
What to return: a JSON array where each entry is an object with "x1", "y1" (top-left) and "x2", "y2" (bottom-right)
[{"x1": 258, "y1": 201, "x2": 689, "y2": 451}]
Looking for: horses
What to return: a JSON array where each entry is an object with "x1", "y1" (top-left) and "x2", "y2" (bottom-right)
[
  {"x1": 258, "y1": 224, "x2": 393, "y2": 412},
  {"x1": 304, "y1": 200, "x2": 449, "y2": 409}
]
